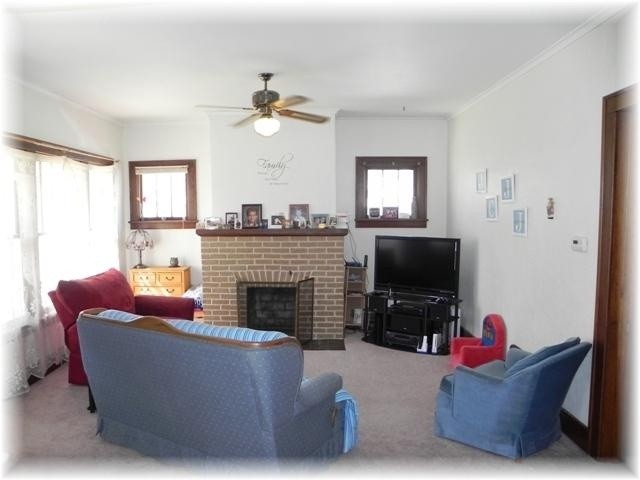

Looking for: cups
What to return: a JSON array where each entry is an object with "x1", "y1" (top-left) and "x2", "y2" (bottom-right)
[
  {"x1": 170, "y1": 258, "x2": 178, "y2": 266},
  {"x1": 351, "y1": 309, "x2": 362, "y2": 323}
]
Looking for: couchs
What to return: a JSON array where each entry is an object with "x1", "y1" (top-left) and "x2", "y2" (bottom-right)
[
  {"x1": 70, "y1": 301, "x2": 359, "y2": 475},
  {"x1": 45, "y1": 268, "x2": 198, "y2": 389}
]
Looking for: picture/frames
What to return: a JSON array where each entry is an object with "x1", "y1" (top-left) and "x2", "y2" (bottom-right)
[
  {"x1": 267, "y1": 212, "x2": 288, "y2": 230},
  {"x1": 485, "y1": 195, "x2": 501, "y2": 222},
  {"x1": 511, "y1": 207, "x2": 529, "y2": 237},
  {"x1": 311, "y1": 211, "x2": 329, "y2": 228},
  {"x1": 224, "y1": 212, "x2": 241, "y2": 230},
  {"x1": 382, "y1": 206, "x2": 399, "y2": 220},
  {"x1": 475, "y1": 166, "x2": 490, "y2": 196},
  {"x1": 240, "y1": 202, "x2": 265, "y2": 229},
  {"x1": 498, "y1": 174, "x2": 516, "y2": 203},
  {"x1": 288, "y1": 202, "x2": 311, "y2": 229}
]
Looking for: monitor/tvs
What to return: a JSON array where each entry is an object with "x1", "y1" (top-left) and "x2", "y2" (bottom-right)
[{"x1": 374, "y1": 235, "x2": 460, "y2": 302}]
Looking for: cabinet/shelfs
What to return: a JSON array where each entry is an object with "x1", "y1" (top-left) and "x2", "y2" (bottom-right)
[
  {"x1": 345, "y1": 262, "x2": 369, "y2": 327},
  {"x1": 358, "y1": 289, "x2": 461, "y2": 357},
  {"x1": 124, "y1": 264, "x2": 193, "y2": 299}
]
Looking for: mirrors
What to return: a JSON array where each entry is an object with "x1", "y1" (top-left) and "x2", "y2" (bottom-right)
[
  {"x1": 353, "y1": 153, "x2": 431, "y2": 232},
  {"x1": 126, "y1": 158, "x2": 199, "y2": 233}
]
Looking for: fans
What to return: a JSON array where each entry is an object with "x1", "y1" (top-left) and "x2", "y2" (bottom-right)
[{"x1": 192, "y1": 69, "x2": 333, "y2": 131}]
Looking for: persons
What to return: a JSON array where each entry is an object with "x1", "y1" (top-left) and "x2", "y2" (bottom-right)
[
  {"x1": 243, "y1": 207, "x2": 260, "y2": 228},
  {"x1": 294, "y1": 208, "x2": 307, "y2": 228}
]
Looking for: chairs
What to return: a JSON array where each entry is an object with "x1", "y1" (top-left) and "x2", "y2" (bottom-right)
[
  {"x1": 446, "y1": 307, "x2": 508, "y2": 367},
  {"x1": 434, "y1": 335, "x2": 595, "y2": 464}
]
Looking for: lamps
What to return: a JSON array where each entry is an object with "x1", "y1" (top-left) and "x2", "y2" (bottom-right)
[
  {"x1": 123, "y1": 226, "x2": 156, "y2": 270},
  {"x1": 246, "y1": 111, "x2": 286, "y2": 139}
]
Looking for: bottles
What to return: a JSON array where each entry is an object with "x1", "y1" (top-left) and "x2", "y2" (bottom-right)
[{"x1": 411, "y1": 194, "x2": 418, "y2": 219}]
